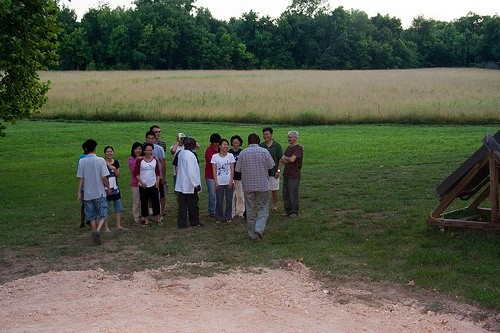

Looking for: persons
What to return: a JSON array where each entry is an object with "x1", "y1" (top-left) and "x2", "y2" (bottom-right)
[
  {"x1": 211, "y1": 139, "x2": 236, "y2": 223},
  {"x1": 281, "y1": 130, "x2": 303, "y2": 218},
  {"x1": 205, "y1": 133, "x2": 221, "y2": 216},
  {"x1": 103, "y1": 146, "x2": 128, "y2": 232},
  {"x1": 227, "y1": 135, "x2": 245, "y2": 219},
  {"x1": 145, "y1": 131, "x2": 166, "y2": 220},
  {"x1": 150, "y1": 125, "x2": 167, "y2": 215},
  {"x1": 235, "y1": 134, "x2": 276, "y2": 241},
  {"x1": 133, "y1": 143, "x2": 163, "y2": 227},
  {"x1": 76, "y1": 139, "x2": 112, "y2": 245},
  {"x1": 174, "y1": 137, "x2": 204, "y2": 228},
  {"x1": 258, "y1": 127, "x2": 283, "y2": 212},
  {"x1": 170, "y1": 133, "x2": 200, "y2": 205},
  {"x1": 128, "y1": 142, "x2": 159, "y2": 223}
]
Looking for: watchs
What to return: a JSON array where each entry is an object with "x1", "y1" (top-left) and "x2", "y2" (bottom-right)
[{"x1": 277, "y1": 170, "x2": 280, "y2": 172}]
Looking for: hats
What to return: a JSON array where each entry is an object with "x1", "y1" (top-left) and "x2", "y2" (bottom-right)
[{"x1": 177, "y1": 133, "x2": 187, "y2": 138}]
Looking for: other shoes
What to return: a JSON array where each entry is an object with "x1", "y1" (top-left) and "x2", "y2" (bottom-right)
[
  {"x1": 216, "y1": 220, "x2": 222, "y2": 223},
  {"x1": 226, "y1": 219, "x2": 233, "y2": 223},
  {"x1": 157, "y1": 221, "x2": 162, "y2": 225},
  {"x1": 280, "y1": 212, "x2": 297, "y2": 218},
  {"x1": 250, "y1": 231, "x2": 262, "y2": 242},
  {"x1": 92, "y1": 231, "x2": 101, "y2": 245},
  {"x1": 272, "y1": 207, "x2": 277, "y2": 213},
  {"x1": 142, "y1": 224, "x2": 148, "y2": 227}
]
